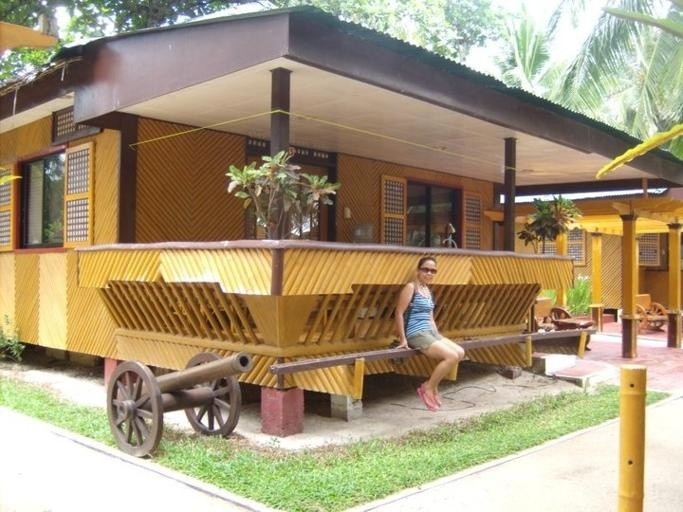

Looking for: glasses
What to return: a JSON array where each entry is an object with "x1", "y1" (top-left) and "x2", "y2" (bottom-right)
[{"x1": 419, "y1": 267, "x2": 438, "y2": 274}]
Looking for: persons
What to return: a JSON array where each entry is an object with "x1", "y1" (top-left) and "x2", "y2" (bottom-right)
[{"x1": 395, "y1": 257, "x2": 465, "y2": 411}]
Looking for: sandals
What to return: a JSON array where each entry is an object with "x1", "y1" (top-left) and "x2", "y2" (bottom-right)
[{"x1": 416, "y1": 384, "x2": 443, "y2": 412}]
